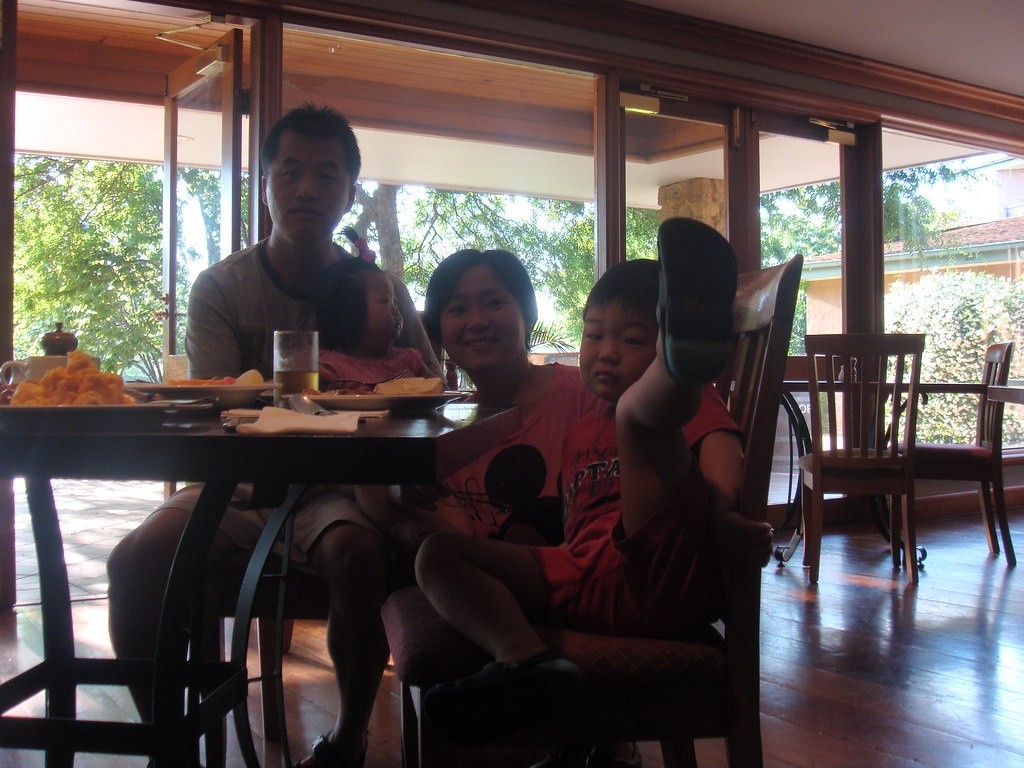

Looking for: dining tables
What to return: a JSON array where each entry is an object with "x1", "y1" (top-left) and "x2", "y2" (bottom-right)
[{"x1": 0, "y1": 395, "x2": 487, "y2": 768}]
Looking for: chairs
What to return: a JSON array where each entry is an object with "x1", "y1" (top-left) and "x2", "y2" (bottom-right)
[
  {"x1": 378, "y1": 254, "x2": 804, "y2": 767},
  {"x1": 262, "y1": 309, "x2": 459, "y2": 741},
  {"x1": 794, "y1": 331, "x2": 927, "y2": 584},
  {"x1": 886, "y1": 339, "x2": 1017, "y2": 567}
]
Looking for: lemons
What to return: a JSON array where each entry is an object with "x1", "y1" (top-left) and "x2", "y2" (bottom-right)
[{"x1": 235, "y1": 369, "x2": 264, "y2": 385}]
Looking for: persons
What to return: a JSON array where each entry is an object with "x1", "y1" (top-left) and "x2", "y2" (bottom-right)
[
  {"x1": 412, "y1": 217, "x2": 774, "y2": 746},
  {"x1": 297, "y1": 225, "x2": 446, "y2": 556},
  {"x1": 109, "y1": 99, "x2": 448, "y2": 768},
  {"x1": 385, "y1": 247, "x2": 641, "y2": 768}
]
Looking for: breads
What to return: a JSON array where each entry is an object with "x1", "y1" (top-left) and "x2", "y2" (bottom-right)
[{"x1": 375, "y1": 376, "x2": 444, "y2": 395}]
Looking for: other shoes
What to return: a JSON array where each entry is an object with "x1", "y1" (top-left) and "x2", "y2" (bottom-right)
[
  {"x1": 424, "y1": 652, "x2": 583, "y2": 740},
  {"x1": 585, "y1": 741, "x2": 643, "y2": 768},
  {"x1": 655, "y1": 218, "x2": 737, "y2": 384}
]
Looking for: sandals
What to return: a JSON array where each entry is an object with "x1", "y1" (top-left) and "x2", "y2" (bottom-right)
[{"x1": 286, "y1": 727, "x2": 368, "y2": 768}]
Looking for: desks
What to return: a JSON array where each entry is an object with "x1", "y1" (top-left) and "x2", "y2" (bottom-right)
[{"x1": 767, "y1": 382, "x2": 987, "y2": 567}]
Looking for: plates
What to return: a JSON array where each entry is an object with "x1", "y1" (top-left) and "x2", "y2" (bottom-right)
[
  {"x1": 123, "y1": 382, "x2": 282, "y2": 407},
  {"x1": 278, "y1": 392, "x2": 474, "y2": 415},
  {"x1": 0, "y1": 398, "x2": 215, "y2": 432}
]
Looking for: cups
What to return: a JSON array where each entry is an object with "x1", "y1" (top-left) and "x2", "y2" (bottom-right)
[
  {"x1": 0, "y1": 356, "x2": 101, "y2": 394},
  {"x1": 272, "y1": 329, "x2": 321, "y2": 410},
  {"x1": 162, "y1": 353, "x2": 191, "y2": 383}
]
